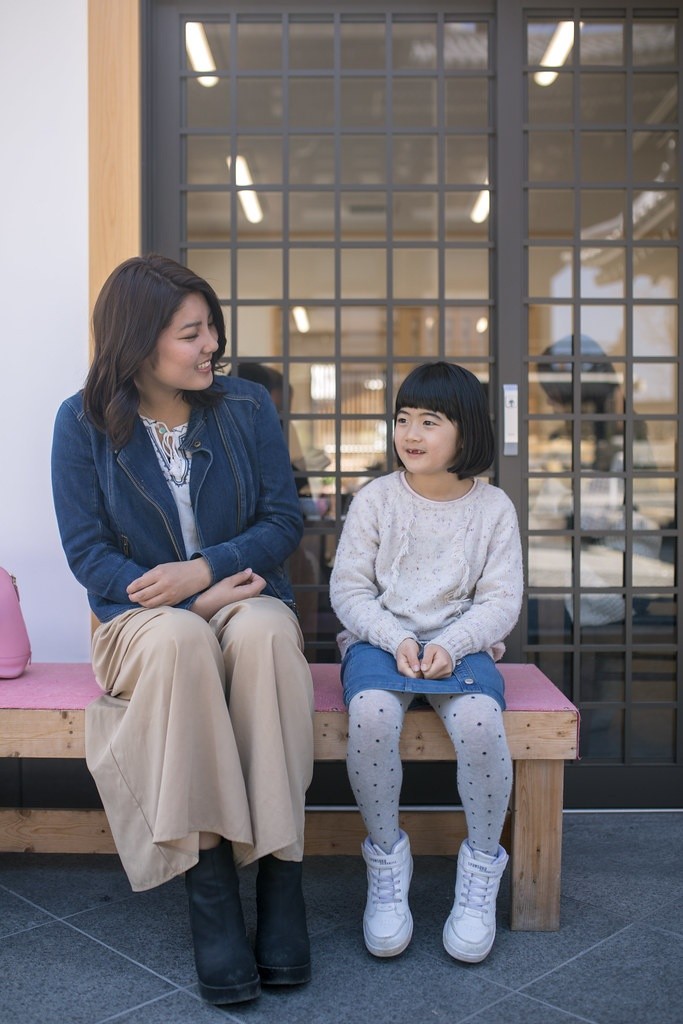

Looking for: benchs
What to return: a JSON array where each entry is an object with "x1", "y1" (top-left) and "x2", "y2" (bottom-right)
[{"x1": 0, "y1": 662, "x2": 582, "y2": 932}]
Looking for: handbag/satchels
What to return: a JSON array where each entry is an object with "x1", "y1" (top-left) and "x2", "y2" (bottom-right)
[{"x1": 0, "y1": 567, "x2": 31, "y2": 681}]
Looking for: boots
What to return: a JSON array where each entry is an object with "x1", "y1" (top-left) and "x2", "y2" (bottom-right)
[
  {"x1": 256, "y1": 853, "x2": 312, "y2": 985},
  {"x1": 186, "y1": 840, "x2": 261, "y2": 1006}
]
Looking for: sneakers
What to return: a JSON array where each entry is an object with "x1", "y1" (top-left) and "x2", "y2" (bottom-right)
[
  {"x1": 361, "y1": 828, "x2": 413, "y2": 958},
  {"x1": 443, "y1": 838, "x2": 508, "y2": 962}
]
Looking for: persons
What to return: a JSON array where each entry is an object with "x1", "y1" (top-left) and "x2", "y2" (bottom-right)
[
  {"x1": 227, "y1": 363, "x2": 308, "y2": 495},
  {"x1": 528, "y1": 334, "x2": 662, "y2": 643},
  {"x1": 51, "y1": 255, "x2": 314, "y2": 1004},
  {"x1": 330, "y1": 362, "x2": 524, "y2": 962}
]
